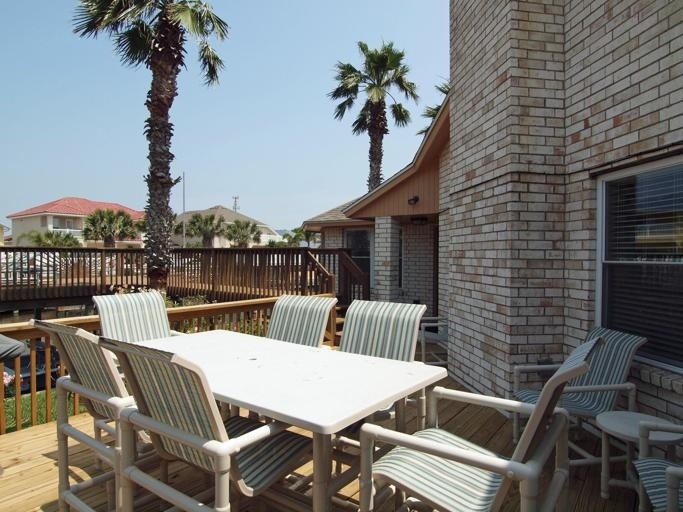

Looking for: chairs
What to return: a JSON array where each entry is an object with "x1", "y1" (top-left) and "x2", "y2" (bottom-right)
[
  {"x1": 338, "y1": 299, "x2": 427, "y2": 361},
  {"x1": 266, "y1": 294, "x2": 338, "y2": 347},
  {"x1": 513, "y1": 326, "x2": 648, "y2": 442},
  {"x1": 96, "y1": 336, "x2": 312, "y2": 512},
  {"x1": 358, "y1": 338, "x2": 608, "y2": 512},
  {"x1": 92, "y1": 291, "x2": 185, "y2": 341},
  {"x1": 637, "y1": 420, "x2": 683, "y2": 512},
  {"x1": 29, "y1": 318, "x2": 152, "y2": 512}
]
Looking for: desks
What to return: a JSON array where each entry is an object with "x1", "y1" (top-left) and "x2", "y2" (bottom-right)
[
  {"x1": 595, "y1": 411, "x2": 683, "y2": 512},
  {"x1": 135, "y1": 329, "x2": 448, "y2": 512}
]
